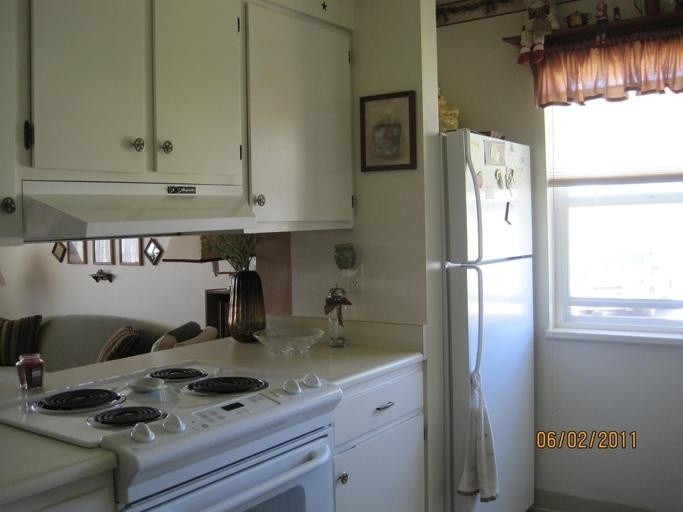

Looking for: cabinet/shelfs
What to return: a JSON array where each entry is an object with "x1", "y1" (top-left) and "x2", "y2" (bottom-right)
[
  {"x1": 326, "y1": 352, "x2": 426, "y2": 512},
  {"x1": 0, "y1": 463, "x2": 115, "y2": 511},
  {"x1": 240, "y1": 0, "x2": 354, "y2": 226},
  {"x1": 0, "y1": 0, "x2": 244, "y2": 190}
]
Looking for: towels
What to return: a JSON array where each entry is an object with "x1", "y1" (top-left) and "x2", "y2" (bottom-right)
[{"x1": 456, "y1": 370, "x2": 500, "y2": 503}]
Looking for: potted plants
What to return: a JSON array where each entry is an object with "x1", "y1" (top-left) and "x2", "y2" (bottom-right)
[{"x1": 194, "y1": 231, "x2": 268, "y2": 344}]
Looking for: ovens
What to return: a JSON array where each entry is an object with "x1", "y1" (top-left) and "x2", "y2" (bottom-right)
[{"x1": 131, "y1": 430, "x2": 334, "y2": 511}]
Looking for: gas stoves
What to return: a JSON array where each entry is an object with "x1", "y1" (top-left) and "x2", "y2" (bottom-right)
[{"x1": 0, "y1": 358, "x2": 343, "y2": 505}]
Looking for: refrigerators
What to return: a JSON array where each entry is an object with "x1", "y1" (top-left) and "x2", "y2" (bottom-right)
[{"x1": 442, "y1": 127, "x2": 538, "y2": 511}]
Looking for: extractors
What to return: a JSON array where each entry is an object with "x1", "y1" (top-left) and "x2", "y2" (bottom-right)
[{"x1": 20, "y1": 179, "x2": 255, "y2": 243}]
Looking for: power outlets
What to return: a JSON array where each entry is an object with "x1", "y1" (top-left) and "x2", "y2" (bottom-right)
[{"x1": 347, "y1": 263, "x2": 365, "y2": 294}]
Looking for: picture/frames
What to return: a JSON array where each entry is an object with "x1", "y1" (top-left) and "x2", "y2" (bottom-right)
[
  {"x1": 143, "y1": 238, "x2": 163, "y2": 265},
  {"x1": 51, "y1": 241, "x2": 65, "y2": 263},
  {"x1": 64, "y1": 239, "x2": 87, "y2": 265},
  {"x1": 92, "y1": 239, "x2": 114, "y2": 266},
  {"x1": 117, "y1": 237, "x2": 143, "y2": 266},
  {"x1": 358, "y1": 89, "x2": 416, "y2": 175}
]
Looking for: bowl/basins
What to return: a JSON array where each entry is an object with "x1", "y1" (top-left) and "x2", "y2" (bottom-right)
[{"x1": 252, "y1": 325, "x2": 326, "y2": 352}]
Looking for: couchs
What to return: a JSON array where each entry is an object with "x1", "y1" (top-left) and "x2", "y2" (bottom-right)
[{"x1": 0, "y1": 310, "x2": 218, "y2": 383}]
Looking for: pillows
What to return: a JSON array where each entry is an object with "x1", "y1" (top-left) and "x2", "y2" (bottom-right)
[
  {"x1": 0, "y1": 314, "x2": 43, "y2": 368},
  {"x1": 94, "y1": 323, "x2": 141, "y2": 363}
]
